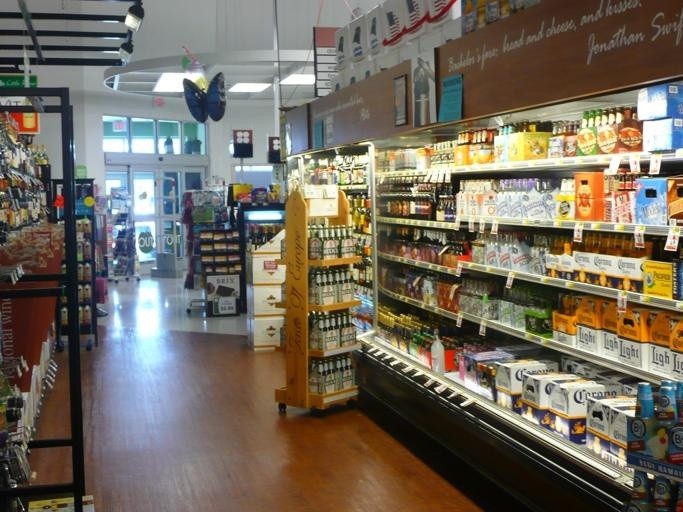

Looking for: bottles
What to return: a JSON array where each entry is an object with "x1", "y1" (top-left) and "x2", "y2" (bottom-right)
[
  {"x1": 347, "y1": 195, "x2": 369, "y2": 227},
  {"x1": 562, "y1": 233, "x2": 646, "y2": 257},
  {"x1": 27, "y1": 144, "x2": 49, "y2": 181},
  {"x1": 306, "y1": 225, "x2": 372, "y2": 395},
  {"x1": 602, "y1": 168, "x2": 642, "y2": 190},
  {"x1": 378, "y1": 116, "x2": 560, "y2": 376},
  {"x1": 563, "y1": 103, "x2": 642, "y2": 132},
  {"x1": 0, "y1": 354, "x2": 30, "y2": 434},
  {"x1": 305, "y1": 155, "x2": 371, "y2": 185},
  {"x1": 628, "y1": 374, "x2": 683, "y2": 511},
  {"x1": 249, "y1": 224, "x2": 280, "y2": 246}
]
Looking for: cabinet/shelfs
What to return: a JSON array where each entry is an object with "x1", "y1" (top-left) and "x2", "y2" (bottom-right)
[
  {"x1": 354, "y1": 76, "x2": 681, "y2": 512},
  {"x1": 275, "y1": 188, "x2": 361, "y2": 414}
]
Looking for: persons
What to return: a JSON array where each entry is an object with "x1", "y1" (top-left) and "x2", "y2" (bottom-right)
[{"x1": 112, "y1": 213, "x2": 135, "y2": 275}]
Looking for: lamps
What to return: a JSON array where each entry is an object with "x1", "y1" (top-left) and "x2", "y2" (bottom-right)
[
  {"x1": 123, "y1": 0, "x2": 143, "y2": 33},
  {"x1": 117, "y1": 30, "x2": 133, "y2": 63}
]
